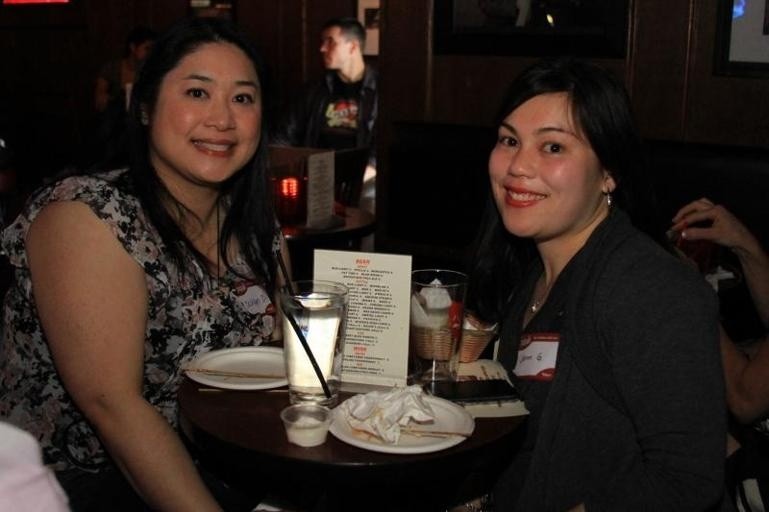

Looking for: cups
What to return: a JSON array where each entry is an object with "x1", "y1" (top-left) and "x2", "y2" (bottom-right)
[
  {"x1": 281, "y1": 404, "x2": 335, "y2": 447},
  {"x1": 279, "y1": 279, "x2": 350, "y2": 406},
  {"x1": 412, "y1": 268, "x2": 466, "y2": 386}
]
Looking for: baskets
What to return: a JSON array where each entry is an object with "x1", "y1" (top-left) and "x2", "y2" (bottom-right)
[{"x1": 412, "y1": 326, "x2": 493, "y2": 363}]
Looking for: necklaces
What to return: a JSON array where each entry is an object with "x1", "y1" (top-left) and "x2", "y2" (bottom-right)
[{"x1": 530, "y1": 280, "x2": 551, "y2": 313}]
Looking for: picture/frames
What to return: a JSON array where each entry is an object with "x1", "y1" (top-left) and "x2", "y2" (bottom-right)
[
  {"x1": 432, "y1": 0, "x2": 635, "y2": 64},
  {"x1": 711, "y1": 1, "x2": 769, "y2": 81}
]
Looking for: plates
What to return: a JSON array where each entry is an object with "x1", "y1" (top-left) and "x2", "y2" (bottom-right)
[
  {"x1": 328, "y1": 387, "x2": 489, "y2": 453},
  {"x1": 184, "y1": 346, "x2": 288, "y2": 391}
]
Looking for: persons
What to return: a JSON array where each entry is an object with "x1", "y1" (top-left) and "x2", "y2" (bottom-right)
[
  {"x1": 270, "y1": 15, "x2": 379, "y2": 254},
  {"x1": 673, "y1": 197, "x2": 769, "y2": 512},
  {"x1": 448, "y1": 57, "x2": 727, "y2": 512},
  {"x1": 1, "y1": 15, "x2": 293, "y2": 511},
  {"x1": 93, "y1": 25, "x2": 159, "y2": 115}
]
